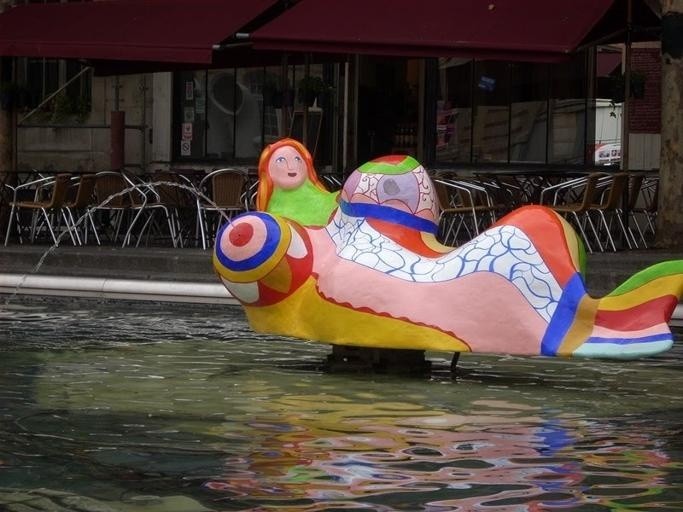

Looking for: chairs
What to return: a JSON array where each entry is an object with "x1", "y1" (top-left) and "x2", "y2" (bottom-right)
[
  {"x1": 318, "y1": 172, "x2": 347, "y2": 193},
  {"x1": 429, "y1": 170, "x2": 658, "y2": 255},
  {"x1": 0, "y1": 166, "x2": 259, "y2": 251}
]
